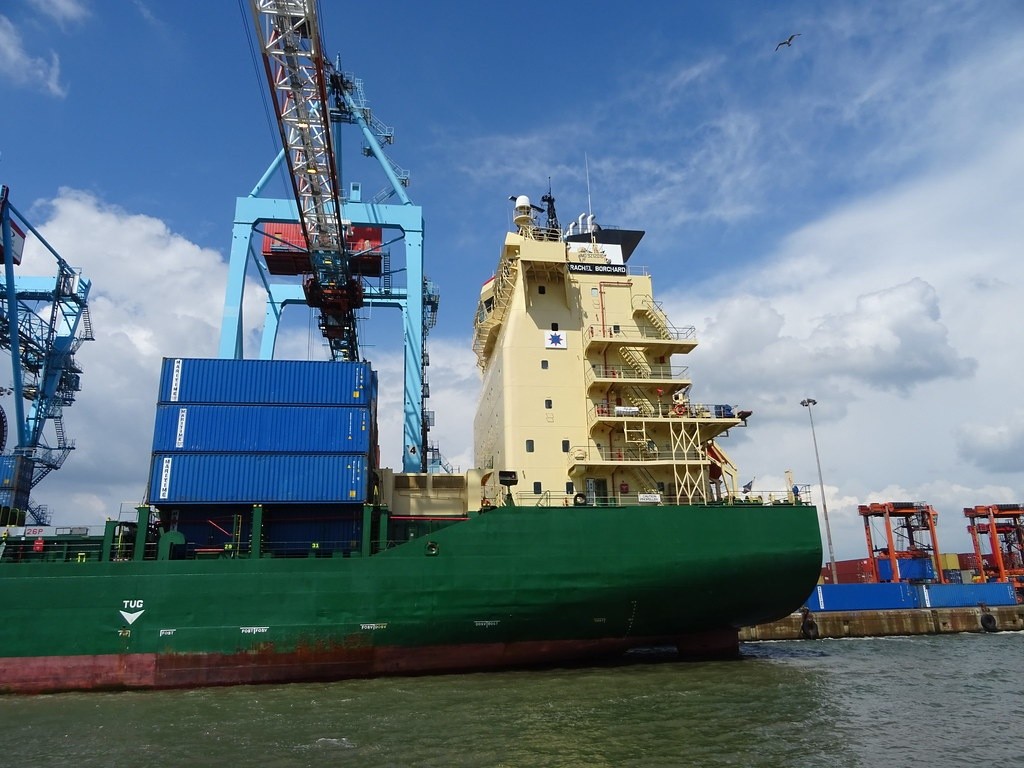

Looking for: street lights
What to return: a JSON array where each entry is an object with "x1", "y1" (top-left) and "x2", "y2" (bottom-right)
[{"x1": 800, "y1": 397, "x2": 838, "y2": 583}]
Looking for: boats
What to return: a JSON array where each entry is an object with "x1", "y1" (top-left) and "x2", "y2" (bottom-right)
[{"x1": 1, "y1": 503, "x2": 824, "y2": 695}]
